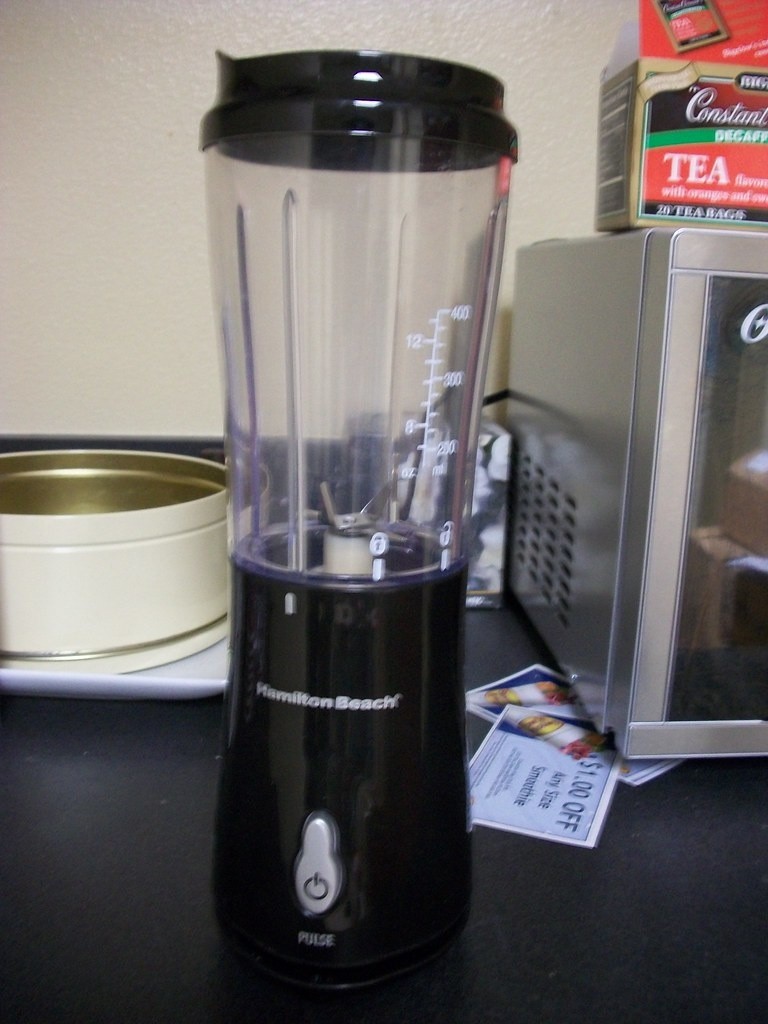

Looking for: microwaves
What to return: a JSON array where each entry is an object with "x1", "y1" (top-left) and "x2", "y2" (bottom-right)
[{"x1": 506, "y1": 225, "x2": 768, "y2": 765}]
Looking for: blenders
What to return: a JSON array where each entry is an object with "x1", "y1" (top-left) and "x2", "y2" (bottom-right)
[{"x1": 197, "y1": 49, "x2": 521, "y2": 1003}]
